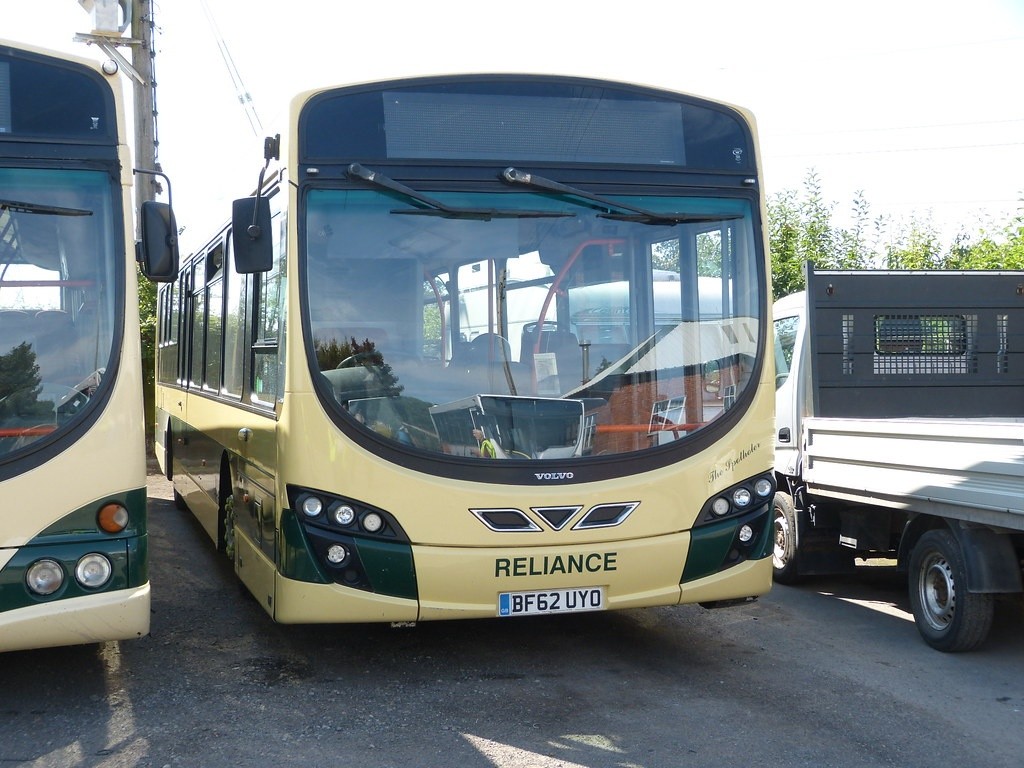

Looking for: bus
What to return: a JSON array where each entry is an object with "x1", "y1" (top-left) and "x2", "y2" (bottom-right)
[
  {"x1": 1, "y1": 38, "x2": 179, "y2": 651},
  {"x1": 152, "y1": 74, "x2": 776, "y2": 624}
]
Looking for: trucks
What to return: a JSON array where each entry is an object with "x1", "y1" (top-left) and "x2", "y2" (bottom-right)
[
  {"x1": 444, "y1": 241, "x2": 790, "y2": 393},
  {"x1": 770, "y1": 259, "x2": 1024, "y2": 653}
]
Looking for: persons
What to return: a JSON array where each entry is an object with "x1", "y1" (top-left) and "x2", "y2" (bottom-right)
[
  {"x1": 473, "y1": 430, "x2": 496, "y2": 459},
  {"x1": 355, "y1": 409, "x2": 363, "y2": 423}
]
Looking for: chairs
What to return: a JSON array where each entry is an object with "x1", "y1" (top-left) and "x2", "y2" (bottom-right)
[
  {"x1": 519, "y1": 320, "x2": 570, "y2": 365},
  {"x1": 0, "y1": 310, "x2": 33, "y2": 355},
  {"x1": 314, "y1": 327, "x2": 389, "y2": 371},
  {"x1": 35, "y1": 310, "x2": 80, "y2": 380}
]
